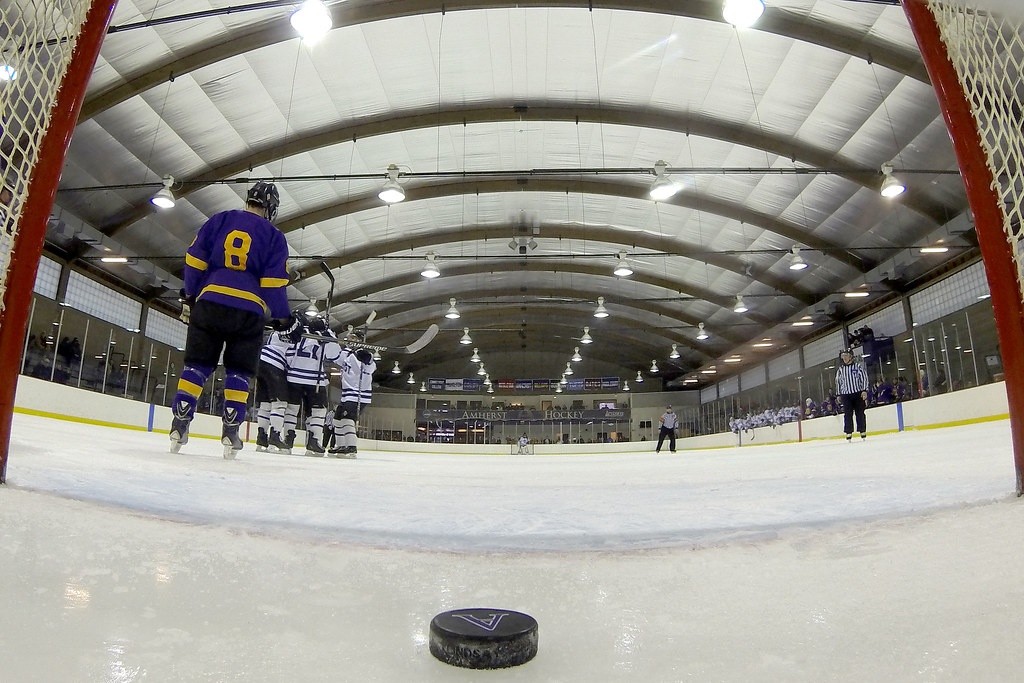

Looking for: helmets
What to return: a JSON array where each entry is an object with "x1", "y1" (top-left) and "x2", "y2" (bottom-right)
[
  {"x1": 839, "y1": 348, "x2": 854, "y2": 356},
  {"x1": 246, "y1": 182, "x2": 279, "y2": 222},
  {"x1": 347, "y1": 330, "x2": 366, "y2": 349},
  {"x1": 309, "y1": 319, "x2": 329, "y2": 336},
  {"x1": 667, "y1": 405, "x2": 672, "y2": 409}
]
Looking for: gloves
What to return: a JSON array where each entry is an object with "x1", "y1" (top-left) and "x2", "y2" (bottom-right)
[
  {"x1": 272, "y1": 315, "x2": 303, "y2": 344},
  {"x1": 179, "y1": 288, "x2": 196, "y2": 325},
  {"x1": 356, "y1": 350, "x2": 374, "y2": 365},
  {"x1": 861, "y1": 391, "x2": 868, "y2": 400}
]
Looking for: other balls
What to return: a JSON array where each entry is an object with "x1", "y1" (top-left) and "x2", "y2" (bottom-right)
[{"x1": 428, "y1": 607, "x2": 538, "y2": 671}]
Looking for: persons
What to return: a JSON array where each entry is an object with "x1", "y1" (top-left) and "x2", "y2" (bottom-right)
[
  {"x1": 28, "y1": 331, "x2": 81, "y2": 367},
  {"x1": 477, "y1": 436, "x2": 501, "y2": 444},
  {"x1": 728, "y1": 367, "x2": 964, "y2": 433},
  {"x1": 200, "y1": 392, "x2": 223, "y2": 416},
  {"x1": 505, "y1": 403, "x2": 609, "y2": 410},
  {"x1": 376, "y1": 431, "x2": 422, "y2": 442},
  {"x1": 834, "y1": 349, "x2": 869, "y2": 442},
  {"x1": 168, "y1": 183, "x2": 306, "y2": 460},
  {"x1": 641, "y1": 436, "x2": 646, "y2": 441},
  {"x1": 103, "y1": 360, "x2": 125, "y2": 388},
  {"x1": 656, "y1": 407, "x2": 679, "y2": 453},
  {"x1": 577, "y1": 436, "x2": 613, "y2": 443},
  {"x1": 543, "y1": 436, "x2": 550, "y2": 444},
  {"x1": 518, "y1": 432, "x2": 529, "y2": 452},
  {"x1": 622, "y1": 402, "x2": 628, "y2": 408},
  {"x1": 256, "y1": 312, "x2": 377, "y2": 458}
]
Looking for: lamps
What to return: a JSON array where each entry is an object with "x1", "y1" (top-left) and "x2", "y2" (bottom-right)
[
  {"x1": 650, "y1": 160, "x2": 677, "y2": 201},
  {"x1": 149, "y1": 176, "x2": 176, "y2": 209},
  {"x1": 348, "y1": 325, "x2": 707, "y2": 393},
  {"x1": 594, "y1": 297, "x2": 609, "y2": 317},
  {"x1": 445, "y1": 298, "x2": 461, "y2": 318},
  {"x1": 723, "y1": 1, "x2": 766, "y2": 26},
  {"x1": 510, "y1": 240, "x2": 538, "y2": 250},
  {"x1": 614, "y1": 252, "x2": 633, "y2": 276},
  {"x1": 288, "y1": 0, "x2": 333, "y2": 36},
  {"x1": 420, "y1": 253, "x2": 439, "y2": 279},
  {"x1": 0, "y1": 38, "x2": 20, "y2": 82},
  {"x1": 305, "y1": 298, "x2": 318, "y2": 316},
  {"x1": 377, "y1": 164, "x2": 405, "y2": 203},
  {"x1": 878, "y1": 163, "x2": 908, "y2": 196},
  {"x1": 789, "y1": 245, "x2": 809, "y2": 270},
  {"x1": 732, "y1": 295, "x2": 749, "y2": 313}
]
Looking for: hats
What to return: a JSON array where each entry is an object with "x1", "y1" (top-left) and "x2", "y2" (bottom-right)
[{"x1": 73, "y1": 337, "x2": 78, "y2": 342}]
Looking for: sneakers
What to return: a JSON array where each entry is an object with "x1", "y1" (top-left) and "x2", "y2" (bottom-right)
[
  {"x1": 170, "y1": 401, "x2": 192, "y2": 453},
  {"x1": 256, "y1": 427, "x2": 269, "y2": 453},
  {"x1": 306, "y1": 430, "x2": 325, "y2": 457},
  {"x1": 346, "y1": 446, "x2": 358, "y2": 459},
  {"x1": 268, "y1": 427, "x2": 291, "y2": 454},
  {"x1": 328, "y1": 446, "x2": 346, "y2": 458},
  {"x1": 221, "y1": 406, "x2": 243, "y2": 459},
  {"x1": 282, "y1": 429, "x2": 296, "y2": 448}
]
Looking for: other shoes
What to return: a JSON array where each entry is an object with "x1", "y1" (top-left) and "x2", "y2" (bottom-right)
[
  {"x1": 846, "y1": 433, "x2": 852, "y2": 442},
  {"x1": 656, "y1": 450, "x2": 659, "y2": 454},
  {"x1": 861, "y1": 431, "x2": 866, "y2": 441},
  {"x1": 670, "y1": 450, "x2": 676, "y2": 453}
]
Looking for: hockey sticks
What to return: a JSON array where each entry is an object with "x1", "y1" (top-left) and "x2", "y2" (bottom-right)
[
  {"x1": 316, "y1": 261, "x2": 335, "y2": 395},
  {"x1": 264, "y1": 323, "x2": 440, "y2": 355},
  {"x1": 355, "y1": 310, "x2": 378, "y2": 432}
]
